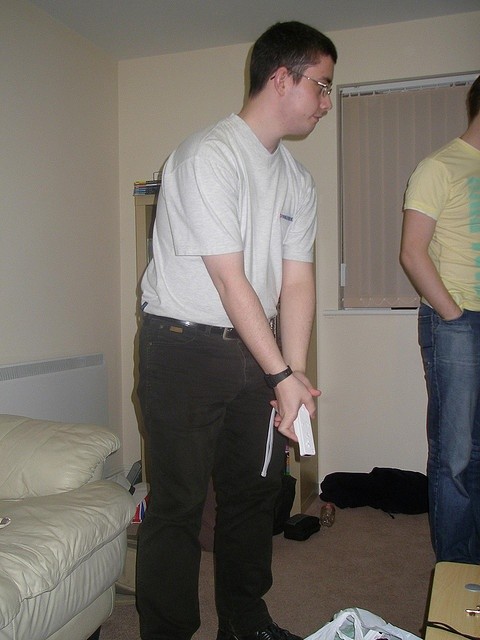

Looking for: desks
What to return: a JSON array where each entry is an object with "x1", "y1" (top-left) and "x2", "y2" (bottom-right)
[{"x1": 425, "y1": 562, "x2": 480, "y2": 639}]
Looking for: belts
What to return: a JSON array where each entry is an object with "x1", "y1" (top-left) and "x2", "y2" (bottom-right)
[{"x1": 146, "y1": 315, "x2": 277, "y2": 341}]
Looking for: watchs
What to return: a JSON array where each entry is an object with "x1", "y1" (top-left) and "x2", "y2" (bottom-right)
[{"x1": 261, "y1": 366, "x2": 293, "y2": 387}]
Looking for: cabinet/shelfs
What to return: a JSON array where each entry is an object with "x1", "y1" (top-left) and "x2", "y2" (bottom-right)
[{"x1": 136, "y1": 190, "x2": 319, "y2": 516}]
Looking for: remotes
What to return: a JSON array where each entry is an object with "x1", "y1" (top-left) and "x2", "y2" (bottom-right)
[{"x1": 292, "y1": 403, "x2": 317, "y2": 457}]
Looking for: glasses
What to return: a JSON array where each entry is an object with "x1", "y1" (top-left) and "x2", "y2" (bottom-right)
[{"x1": 270, "y1": 70, "x2": 332, "y2": 97}]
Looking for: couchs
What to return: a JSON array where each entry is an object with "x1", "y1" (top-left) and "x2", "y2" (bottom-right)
[{"x1": 1, "y1": 416, "x2": 134, "y2": 640}]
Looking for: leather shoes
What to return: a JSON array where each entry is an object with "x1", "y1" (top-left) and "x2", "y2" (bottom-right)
[{"x1": 216, "y1": 622, "x2": 302, "y2": 639}]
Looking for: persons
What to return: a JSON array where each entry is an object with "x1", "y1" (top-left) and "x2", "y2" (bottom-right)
[
  {"x1": 137, "y1": 21, "x2": 338, "y2": 639},
  {"x1": 399, "y1": 75, "x2": 479, "y2": 565}
]
